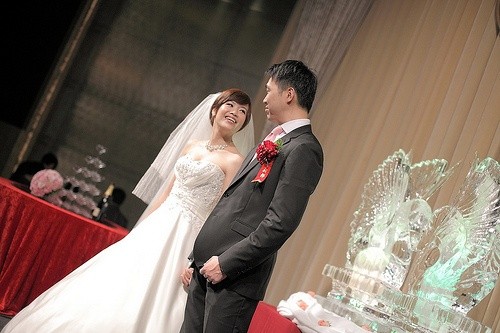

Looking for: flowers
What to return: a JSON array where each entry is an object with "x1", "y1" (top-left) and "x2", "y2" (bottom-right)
[
  {"x1": 29, "y1": 168, "x2": 65, "y2": 207},
  {"x1": 256, "y1": 138, "x2": 284, "y2": 165}
]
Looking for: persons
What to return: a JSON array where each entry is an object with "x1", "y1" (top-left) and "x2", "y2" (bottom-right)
[
  {"x1": 181, "y1": 60, "x2": 324, "y2": 333},
  {"x1": 0, "y1": 89, "x2": 255, "y2": 333},
  {"x1": 11, "y1": 152, "x2": 59, "y2": 184},
  {"x1": 102, "y1": 188, "x2": 128, "y2": 228}
]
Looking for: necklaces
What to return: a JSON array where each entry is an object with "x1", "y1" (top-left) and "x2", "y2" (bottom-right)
[{"x1": 206, "y1": 140, "x2": 233, "y2": 152}]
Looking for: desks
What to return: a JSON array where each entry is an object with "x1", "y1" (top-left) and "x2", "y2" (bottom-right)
[{"x1": 0, "y1": 176, "x2": 131, "y2": 320}]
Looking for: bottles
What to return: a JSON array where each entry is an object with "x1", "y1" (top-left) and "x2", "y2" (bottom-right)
[{"x1": 92, "y1": 184, "x2": 115, "y2": 220}]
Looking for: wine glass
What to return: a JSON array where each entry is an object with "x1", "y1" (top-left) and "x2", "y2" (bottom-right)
[
  {"x1": 51, "y1": 189, "x2": 61, "y2": 206},
  {"x1": 80, "y1": 182, "x2": 89, "y2": 197},
  {"x1": 63, "y1": 191, "x2": 74, "y2": 210},
  {"x1": 82, "y1": 167, "x2": 90, "y2": 183},
  {"x1": 71, "y1": 164, "x2": 81, "y2": 179},
  {"x1": 89, "y1": 200, "x2": 98, "y2": 220},
  {"x1": 90, "y1": 186, "x2": 100, "y2": 200},
  {"x1": 96, "y1": 143, "x2": 105, "y2": 159},
  {"x1": 60, "y1": 172, "x2": 68, "y2": 190},
  {"x1": 85, "y1": 155, "x2": 94, "y2": 170},
  {"x1": 70, "y1": 177, "x2": 79, "y2": 194},
  {"x1": 91, "y1": 172, "x2": 101, "y2": 186},
  {"x1": 95, "y1": 158, "x2": 105, "y2": 173},
  {"x1": 75, "y1": 197, "x2": 88, "y2": 216}
]
